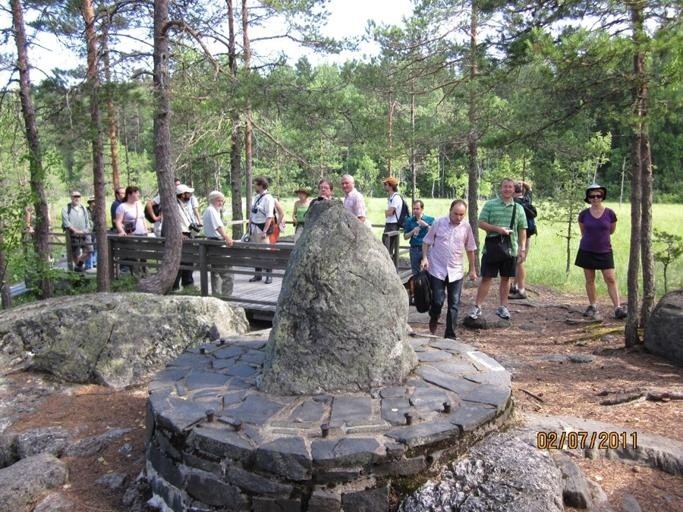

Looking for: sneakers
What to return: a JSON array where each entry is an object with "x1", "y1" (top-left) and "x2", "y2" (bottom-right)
[
  {"x1": 583, "y1": 304, "x2": 597, "y2": 317},
  {"x1": 249, "y1": 275, "x2": 272, "y2": 284},
  {"x1": 469, "y1": 305, "x2": 482, "y2": 320},
  {"x1": 508, "y1": 283, "x2": 527, "y2": 298},
  {"x1": 615, "y1": 306, "x2": 627, "y2": 319},
  {"x1": 495, "y1": 306, "x2": 511, "y2": 320}
]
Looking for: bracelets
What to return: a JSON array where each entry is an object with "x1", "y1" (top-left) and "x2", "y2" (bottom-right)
[{"x1": 520, "y1": 249, "x2": 525, "y2": 252}]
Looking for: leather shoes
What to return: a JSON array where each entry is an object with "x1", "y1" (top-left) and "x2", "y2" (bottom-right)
[{"x1": 429, "y1": 318, "x2": 437, "y2": 334}]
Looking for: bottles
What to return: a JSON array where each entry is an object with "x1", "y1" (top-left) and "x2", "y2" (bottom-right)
[{"x1": 412, "y1": 223, "x2": 421, "y2": 238}]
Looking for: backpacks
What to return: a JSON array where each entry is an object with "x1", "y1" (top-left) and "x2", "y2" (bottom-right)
[{"x1": 390, "y1": 194, "x2": 409, "y2": 228}]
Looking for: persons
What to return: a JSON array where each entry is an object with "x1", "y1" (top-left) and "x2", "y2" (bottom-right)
[
  {"x1": 420, "y1": 200, "x2": 477, "y2": 340},
  {"x1": 404, "y1": 200, "x2": 436, "y2": 305},
  {"x1": 292, "y1": 187, "x2": 313, "y2": 243},
  {"x1": 61, "y1": 176, "x2": 286, "y2": 296},
  {"x1": 342, "y1": 174, "x2": 370, "y2": 228},
  {"x1": 382, "y1": 177, "x2": 404, "y2": 259},
  {"x1": 302, "y1": 178, "x2": 346, "y2": 218},
  {"x1": 574, "y1": 184, "x2": 628, "y2": 318},
  {"x1": 509, "y1": 182, "x2": 537, "y2": 298},
  {"x1": 519, "y1": 181, "x2": 533, "y2": 203},
  {"x1": 468, "y1": 178, "x2": 527, "y2": 320}
]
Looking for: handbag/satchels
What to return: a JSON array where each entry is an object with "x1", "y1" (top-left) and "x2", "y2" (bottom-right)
[
  {"x1": 257, "y1": 223, "x2": 273, "y2": 234},
  {"x1": 144, "y1": 203, "x2": 161, "y2": 223},
  {"x1": 413, "y1": 269, "x2": 433, "y2": 313},
  {"x1": 524, "y1": 205, "x2": 537, "y2": 219},
  {"x1": 124, "y1": 222, "x2": 136, "y2": 235},
  {"x1": 485, "y1": 235, "x2": 513, "y2": 263}
]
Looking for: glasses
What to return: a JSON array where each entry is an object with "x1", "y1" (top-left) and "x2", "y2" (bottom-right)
[{"x1": 588, "y1": 195, "x2": 603, "y2": 198}]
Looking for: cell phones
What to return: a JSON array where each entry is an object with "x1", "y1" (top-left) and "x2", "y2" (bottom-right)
[{"x1": 318, "y1": 197, "x2": 323, "y2": 201}]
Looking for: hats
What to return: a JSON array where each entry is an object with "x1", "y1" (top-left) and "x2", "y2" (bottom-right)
[
  {"x1": 383, "y1": 177, "x2": 398, "y2": 188},
  {"x1": 71, "y1": 191, "x2": 81, "y2": 197},
  {"x1": 87, "y1": 195, "x2": 96, "y2": 204},
  {"x1": 583, "y1": 184, "x2": 607, "y2": 204},
  {"x1": 176, "y1": 184, "x2": 196, "y2": 195},
  {"x1": 294, "y1": 188, "x2": 312, "y2": 197}
]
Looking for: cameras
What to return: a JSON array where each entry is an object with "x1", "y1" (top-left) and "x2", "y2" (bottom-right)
[
  {"x1": 126, "y1": 223, "x2": 135, "y2": 232},
  {"x1": 189, "y1": 224, "x2": 200, "y2": 233},
  {"x1": 514, "y1": 184, "x2": 522, "y2": 193}
]
